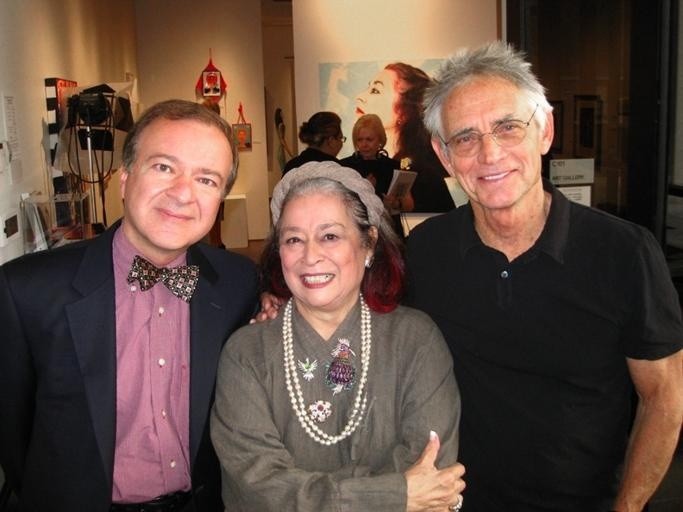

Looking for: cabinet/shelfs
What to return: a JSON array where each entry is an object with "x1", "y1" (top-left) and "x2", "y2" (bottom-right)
[{"x1": 19, "y1": 193, "x2": 90, "y2": 255}]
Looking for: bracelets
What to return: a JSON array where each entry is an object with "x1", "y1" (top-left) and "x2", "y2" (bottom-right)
[{"x1": 398, "y1": 199, "x2": 402, "y2": 209}]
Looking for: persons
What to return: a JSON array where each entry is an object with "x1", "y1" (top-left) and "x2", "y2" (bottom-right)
[
  {"x1": 340, "y1": 114, "x2": 415, "y2": 213},
  {"x1": 355, "y1": 60, "x2": 457, "y2": 213},
  {"x1": 248, "y1": 39, "x2": 683, "y2": 512},
  {"x1": 281, "y1": 112, "x2": 377, "y2": 188},
  {"x1": 208, "y1": 161, "x2": 467, "y2": 512},
  {"x1": 0, "y1": 98, "x2": 263, "y2": 511}
]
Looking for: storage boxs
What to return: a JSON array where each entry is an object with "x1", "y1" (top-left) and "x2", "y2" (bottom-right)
[{"x1": 550, "y1": 159, "x2": 594, "y2": 208}]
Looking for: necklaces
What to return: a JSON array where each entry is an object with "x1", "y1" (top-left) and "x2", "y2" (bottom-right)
[{"x1": 282, "y1": 293, "x2": 371, "y2": 447}]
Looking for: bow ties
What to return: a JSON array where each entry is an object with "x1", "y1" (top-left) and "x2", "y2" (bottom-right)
[{"x1": 127, "y1": 255, "x2": 200, "y2": 304}]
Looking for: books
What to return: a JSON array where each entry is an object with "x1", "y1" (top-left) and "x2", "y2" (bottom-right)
[{"x1": 385, "y1": 170, "x2": 418, "y2": 217}]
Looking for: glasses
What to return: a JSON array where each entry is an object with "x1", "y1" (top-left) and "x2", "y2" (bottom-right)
[
  {"x1": 437, "y1": 103, "x2": 539, "y2": 157},
  {"x1": 335, "y1": 136, "x2": 346, "y2": 143}
]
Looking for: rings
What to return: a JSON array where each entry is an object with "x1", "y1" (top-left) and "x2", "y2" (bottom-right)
[{"x1": 449, "y1": 496, "x2": 463, "y2": 512}]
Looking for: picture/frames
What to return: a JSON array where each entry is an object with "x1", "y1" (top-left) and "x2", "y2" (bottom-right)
[
  {"x1": 548, "y1": 94, "x2": 603, "y2": 171},
  {"x1": 201, "y1": 70, "x2": 221, "y2": 97},
  {"x1": 232, "y1": 123, "x2": 251, "y2": 151}
]
